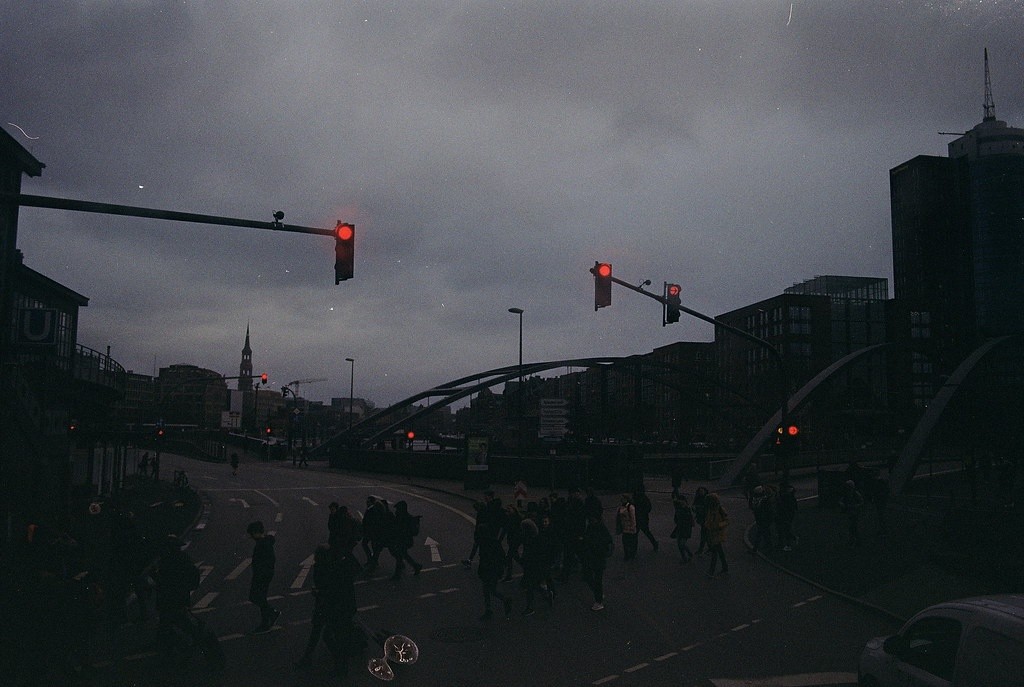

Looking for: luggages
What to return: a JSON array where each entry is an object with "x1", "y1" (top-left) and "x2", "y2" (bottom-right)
[{"x1": 181, "y1": 607, "x2": 225, "y2": 677}]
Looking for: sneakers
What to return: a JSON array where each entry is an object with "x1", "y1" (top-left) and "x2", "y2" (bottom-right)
[
  {"x1": 593, "y1": 593, "x2": 604, "y2": 602},
  {"x1": 592, "y1": 602, "x2": 604, "y2": 610}
]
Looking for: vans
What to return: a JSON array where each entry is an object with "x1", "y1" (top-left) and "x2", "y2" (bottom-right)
[{"x1": 857, "y1": 594, "x2": 1024, "y2": 687}]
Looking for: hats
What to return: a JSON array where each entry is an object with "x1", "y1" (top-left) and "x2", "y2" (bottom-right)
[
  {"x1": 755, "y1": 485, "x2": 763, "y2": 493},
  {"x1": 847, "y1": 480, "x2": 855, "y2": 488}
]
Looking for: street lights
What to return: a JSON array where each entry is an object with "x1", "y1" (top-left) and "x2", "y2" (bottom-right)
[
  {"x1": 344, "y1": 358, "x2": 354, "y2": 448},
  {"x1": 280, "y1": 386, "x2": 297, "y2": 446},
  {"x1": 507, "y1": 307, "x2": 524, "y2": 457}
]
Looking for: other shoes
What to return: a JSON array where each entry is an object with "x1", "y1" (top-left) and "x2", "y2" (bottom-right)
[
  {"x1": 503, "y1": 596, "x2": 511, "y2": 614},
  {"x1": 271, "y1": 610, "x2": 281, "y2": 625},
  {"x1": 505, "y1": 577, "x2": 513, "y2": 583},
  {"x1": 361, "y1": 556, "x2": 423, "y2": 579},
  {"x1": 481, "y1": 608, "x2": 494, "y2": 619},
  {"x1": 522, "y1": 607, "x2": 535, "y2": 616},
  {"x1": 461, "y1": 559, "x2": 473, "y2": 568},
  {"x1": 255, "y1": 623, "x2": 272, "y2": 635},
  {"x1": 544, "y1": 591, "x2": 554, "y2": 607},
  {"x1": 618, "y1": 534, "x2": 800, "y2": 578}
]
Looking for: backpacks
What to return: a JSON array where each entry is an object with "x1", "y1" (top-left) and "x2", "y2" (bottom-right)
[{"x1": 405, "y1": 515, "x2": 422, "y2": 535}]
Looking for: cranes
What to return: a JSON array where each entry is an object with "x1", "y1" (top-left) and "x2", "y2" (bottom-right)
[{"x1": 282, "y1": 376, "x2": 329, "y2": 395}]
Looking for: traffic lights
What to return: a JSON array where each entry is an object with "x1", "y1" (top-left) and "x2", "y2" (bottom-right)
[
  {"x1": 70, "y1": 425, "x2": 75, "y2": 431},
  {"x1": 595, "y1": 263, "x2": 612, "y2": 308},
  {"x1": 158, "y1": 430, "x2": 163, "y2": 436},
  {"x1": 784, "y1": 420, "x2": 799, "y2": 456},
  {"x1": 407, "y1": 431, "x2": 415, "y2": 439},
  {"x1": 334, "y1": 224, "x2": 356, "y2": 281},
  {"x1": 262, "y1": 374, "x2": 267, "y2": 383},
  {"x1": 667, "y1": 284, "x2": 681, "y2": 323},
  {"x1": 267, "y1": 428, "x2": 271, "y2": 433}
]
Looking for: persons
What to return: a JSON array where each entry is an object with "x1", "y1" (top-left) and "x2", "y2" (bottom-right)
[
  {"x1": 58, "y1": 532, "x2": 223, "y2": 678},
  {"x1": 231, "y1": 452, "x2": 239, "y2": 475},
  {"x1": 295, "y1": 496, "x2": 422, "y2": 674},
  {"x1": 461, "y1": 480, "x2": 614, "y2": 616},
  {"x1": 139, "y1": 452, "x2": 151, "y2": 476},
  {"x1": 475, "y1": 443, "x2": 488, "y2": 465},
  {"x1": 619, "y1": 466, "x2": 730, "y2": 577},
  {"x1": 845, "y1": 480, "x2": 864, "y2": 533},
  {"x1": 247, "y1": 521, "x2": 280, "y2": 633},
  {"x1": 299, "y1": 445, "x2": 308, "y2": 466},
  {"x1": 745, "y1": 463, "x2": 800, "y2": 552}
]
